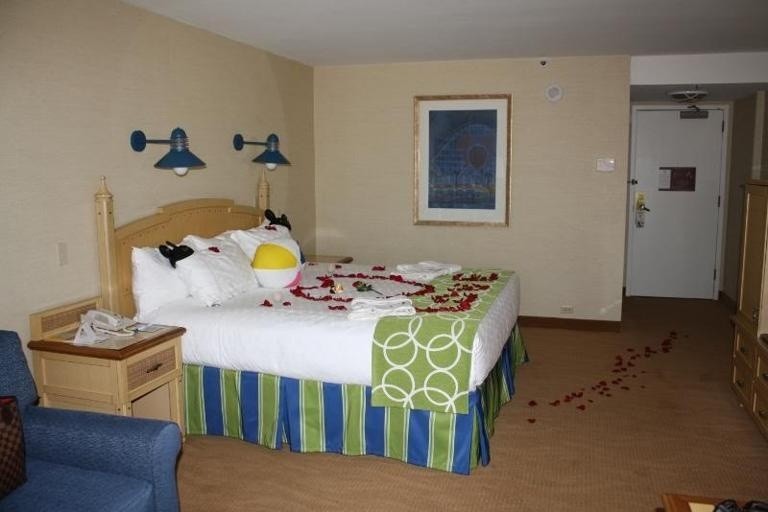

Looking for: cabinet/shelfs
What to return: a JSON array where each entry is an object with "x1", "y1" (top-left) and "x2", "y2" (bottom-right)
[{"x1": 729, "y1": 181, "x2": 768, "y2": 442}]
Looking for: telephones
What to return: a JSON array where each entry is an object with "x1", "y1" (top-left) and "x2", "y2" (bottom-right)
[{"x1": 80, "y1": 310, "x2": 136, "y2": 331}]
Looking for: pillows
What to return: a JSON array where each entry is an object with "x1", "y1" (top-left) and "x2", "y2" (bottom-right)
[{"x1": 127, "y1": 220, "x2": 301, "y2": 322}]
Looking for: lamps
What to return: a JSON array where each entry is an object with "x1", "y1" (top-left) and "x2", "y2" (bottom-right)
[
  {"x1": 129, "y1": 125, "x2": 206, "y2": 176},
  {"x1": 665, "y1": 91, "x2": 708, "y2": 105},
  {"x1": 233, "y1": 132, "x2": 291, "y2": 170}
]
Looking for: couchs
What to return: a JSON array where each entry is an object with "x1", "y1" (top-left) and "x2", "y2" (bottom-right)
[{"x1": 1, "y1": 328, "x2": 183, "y2": 511}]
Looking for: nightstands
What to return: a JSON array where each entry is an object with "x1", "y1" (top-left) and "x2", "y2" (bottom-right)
[{"x1": 29, "y1": 295, "x2": 187, "y2": 447}]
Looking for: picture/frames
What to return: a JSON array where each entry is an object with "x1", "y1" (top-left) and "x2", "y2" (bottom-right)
[{"x1": 411, "y1": 93, "x2": 513, "y2": 231}]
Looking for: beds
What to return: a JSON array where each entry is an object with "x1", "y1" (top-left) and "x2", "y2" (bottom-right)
[{"x1": 91, "y1": 169, "x2": 522, "y2": 476}]
